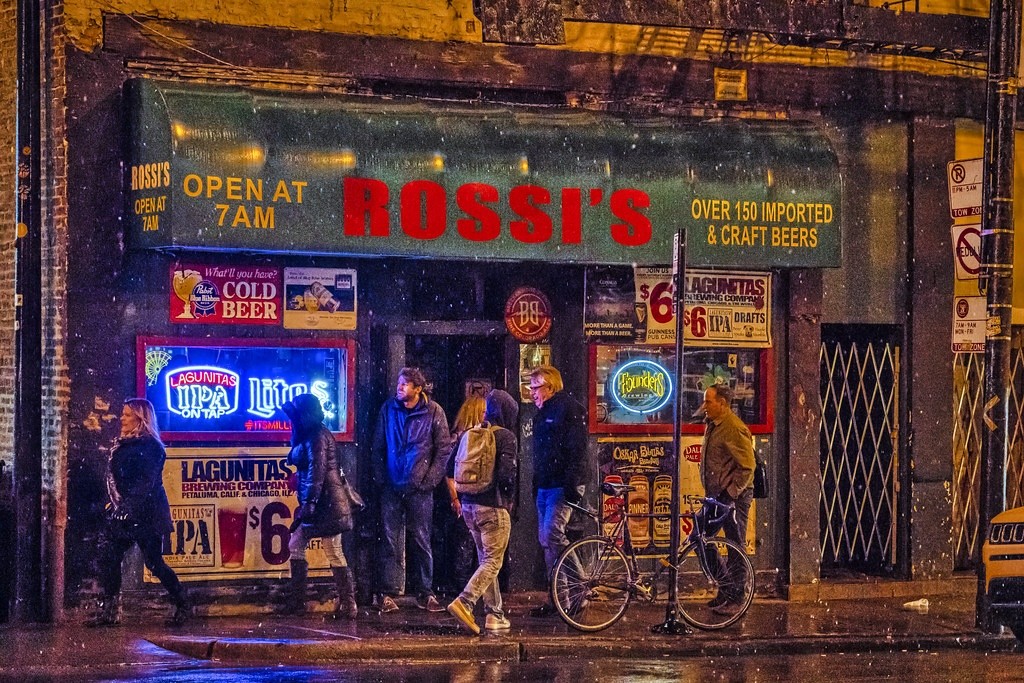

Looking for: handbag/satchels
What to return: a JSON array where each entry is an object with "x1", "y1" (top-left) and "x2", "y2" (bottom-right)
[
  {"x1": 342, "y1": 479, "x2": 365, "y2": 514},
  {"x1": 754, "y1": 462, "x2": 769, "y2": 498}
]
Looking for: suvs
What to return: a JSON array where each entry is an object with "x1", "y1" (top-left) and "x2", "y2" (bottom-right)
[{"x1": 982, "y1": 502, "x2": 1024, "y2": 643}]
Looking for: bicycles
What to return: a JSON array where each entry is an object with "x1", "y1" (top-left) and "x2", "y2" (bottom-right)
[{"x1": 547, "y1": 482, "x2": 756, "y2": 633}]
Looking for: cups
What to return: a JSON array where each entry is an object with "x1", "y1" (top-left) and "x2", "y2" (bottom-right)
[{"x1": 217, "y1": 507, "x2": 247, "y2": 568}]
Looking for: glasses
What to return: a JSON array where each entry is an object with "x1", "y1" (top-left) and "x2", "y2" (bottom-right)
[{"x1": 530, "y1": 385, "x2": 544, "y2": 392}]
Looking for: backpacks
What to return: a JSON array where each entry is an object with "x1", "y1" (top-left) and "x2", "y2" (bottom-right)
[{"x1": 454, "y1": 420, "x2": 507, "y2": 494}]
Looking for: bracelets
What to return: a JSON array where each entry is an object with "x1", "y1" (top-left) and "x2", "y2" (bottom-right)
[{"x1": 452, "y1": 497, "x2": 459, "y2": 502}]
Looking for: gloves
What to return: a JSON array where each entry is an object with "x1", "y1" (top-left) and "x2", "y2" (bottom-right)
[{"x1": 301, "y1": 517, "x2": 316, "y2": 537}]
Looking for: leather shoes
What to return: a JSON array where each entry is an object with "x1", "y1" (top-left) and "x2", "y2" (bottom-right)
[
  {"x1": 528, "y1": 604, "x2": 552, "y2": 617},
  {"x1": 564, "y1": 595, "x2": 588, "y2": 616}
]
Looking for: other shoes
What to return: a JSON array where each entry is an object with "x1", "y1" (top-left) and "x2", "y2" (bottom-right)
[
  {"x1": 173, "y1": 590, "x2": 194, "y2": 626},
  {"x1": 713, "y1": 588, "x2": 747, "y2": 615},
  {"x1": 82, "y1": 595, "x2": 122, "y2": 627},
  {"x1": 707, "y1": 582, "x2": 730, "y2": 608}
]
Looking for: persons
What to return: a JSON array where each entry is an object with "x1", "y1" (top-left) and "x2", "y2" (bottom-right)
[
  {"x1": 371, "y1": 367, "x2": 453, "y2": 613},
  {"x1": 272, "y1": 394, "x2": 359, "y2": 620},
  {"x1": 83, "y1": 399, "x2": 194, "y2": 627},
  {"x1": 446, "y1": 389, "x2": 519, "y2": 635},
  {"x1": 527, "y1": 365, "x2": 594, "y2": 621},
  {"x1": 688, "y1": 384, "x2": 757, "y2": 616}
]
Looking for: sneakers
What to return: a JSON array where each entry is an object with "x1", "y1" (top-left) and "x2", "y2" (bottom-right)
[
  {"x1": 485, "y1": 614, "x2": 511, "y2": 629},
  {"x1": 413, "y1": 592, "x2": 447, "y2": 612},
  {"x1": 377, "y1": 593, "x2": 399, "y2": 612},
  {"x1": 447, "y1": 598, "x2": 481, "y2": 635}
]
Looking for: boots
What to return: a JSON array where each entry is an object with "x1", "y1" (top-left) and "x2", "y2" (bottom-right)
[
  {"x1": 276, "y1": 559, "x2": 308, "y2": 618},
  {"x1": 323, "y1": 566, "x2": 357, "y2": 622}
]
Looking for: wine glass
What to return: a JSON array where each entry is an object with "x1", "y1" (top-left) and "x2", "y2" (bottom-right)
[
  {"x1": 304, "y1": 291, "x2": 320, "y2": 326},
  {"x1": 173, "y1": 275, "x2": 203, "y2": 318}
]
